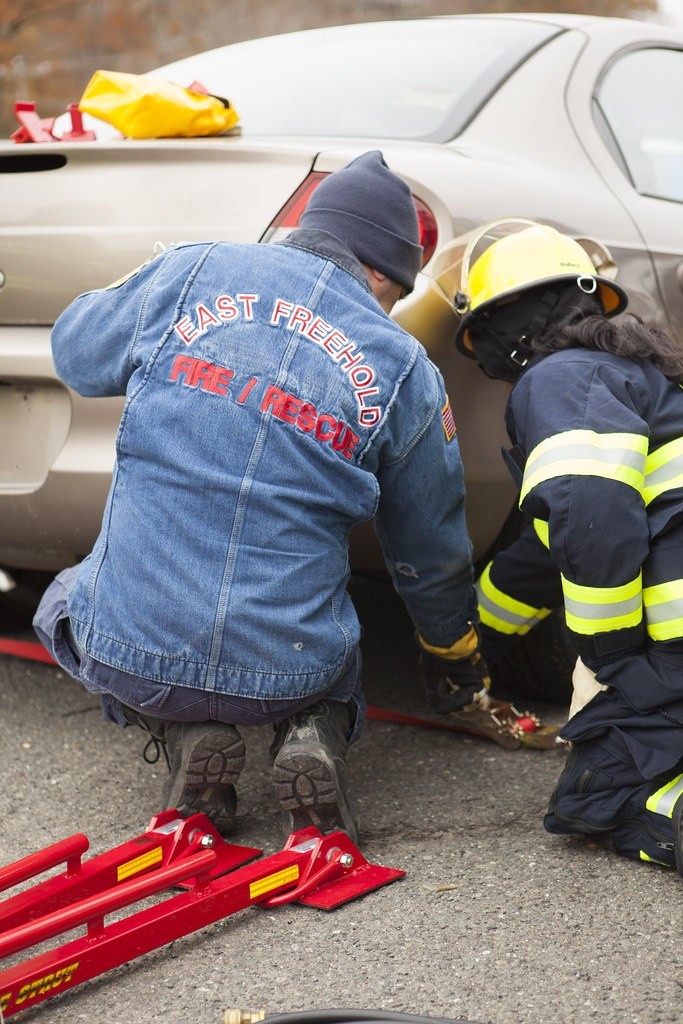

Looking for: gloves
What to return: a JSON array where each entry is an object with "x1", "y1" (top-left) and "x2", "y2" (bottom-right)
[{"x1": 414, "y1": 622, "x2": 491, "y2": 715}]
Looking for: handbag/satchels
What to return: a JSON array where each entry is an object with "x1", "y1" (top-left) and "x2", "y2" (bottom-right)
[{"x1": 77, "y1": 69, "x2": 240, "y2": 139}]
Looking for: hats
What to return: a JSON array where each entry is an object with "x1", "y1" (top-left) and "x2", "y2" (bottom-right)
[{"x1": 299, "y1": 150, "x2": 424, "y2": 295}]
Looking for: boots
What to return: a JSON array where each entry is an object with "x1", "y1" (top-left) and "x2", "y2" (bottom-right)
[
  {"x1": 269, "y1": 697, "x2": 360, "y2": 847},
  {"x1": 123, "y1": 703, "x2": 246, "y2": 836}
]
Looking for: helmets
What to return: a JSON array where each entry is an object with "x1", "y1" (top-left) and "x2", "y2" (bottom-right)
[{"x1": 456, "y1": 230, "x2": 629, "y2": 360}]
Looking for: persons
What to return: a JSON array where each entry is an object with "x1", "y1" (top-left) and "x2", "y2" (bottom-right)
[
  {"x1": 427, "y1": 220, "x2": 683, "y2": 879},
  {"x1": 31, "y1": 150, "x2": 491, "y2": 856}
]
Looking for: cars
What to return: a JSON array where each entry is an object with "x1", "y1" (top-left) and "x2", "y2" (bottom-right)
[{"x1": 0, "y1": 11, "x2": 683, "y2": 578}]
